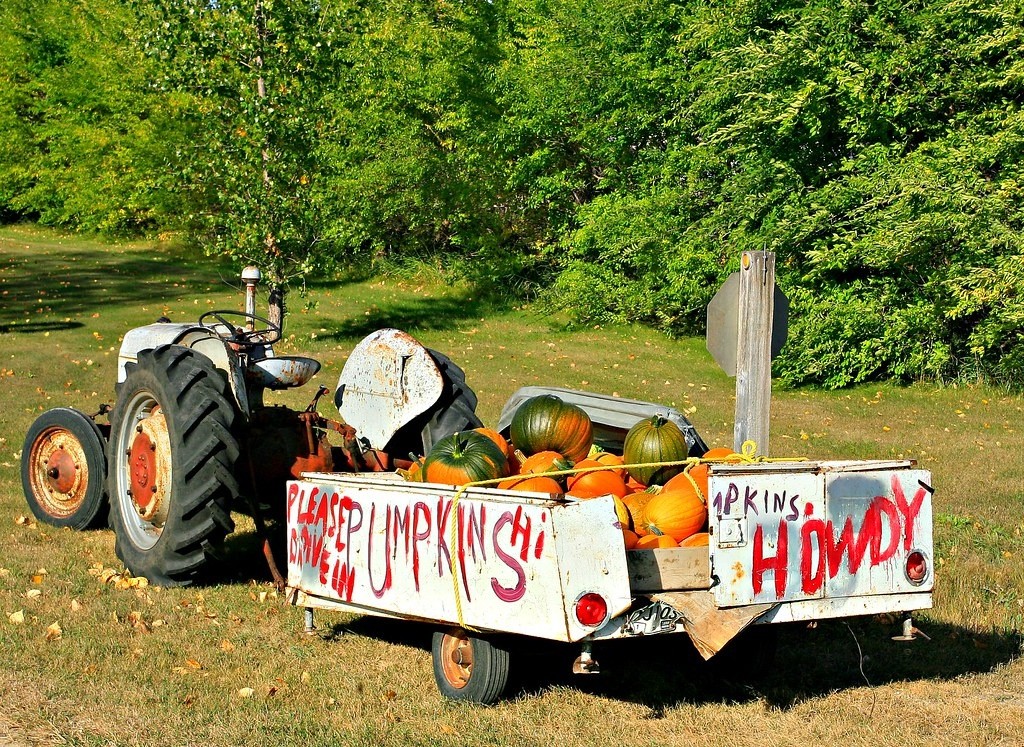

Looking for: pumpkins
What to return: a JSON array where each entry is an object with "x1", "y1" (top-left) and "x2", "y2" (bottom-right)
[{"x1": 406, "y1": 393, "x2": 744, "y2": 548}]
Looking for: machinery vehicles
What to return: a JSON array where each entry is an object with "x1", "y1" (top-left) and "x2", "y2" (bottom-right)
[{"x1": 22, "y1": 263, "x2": 936, "y2": 704}]
